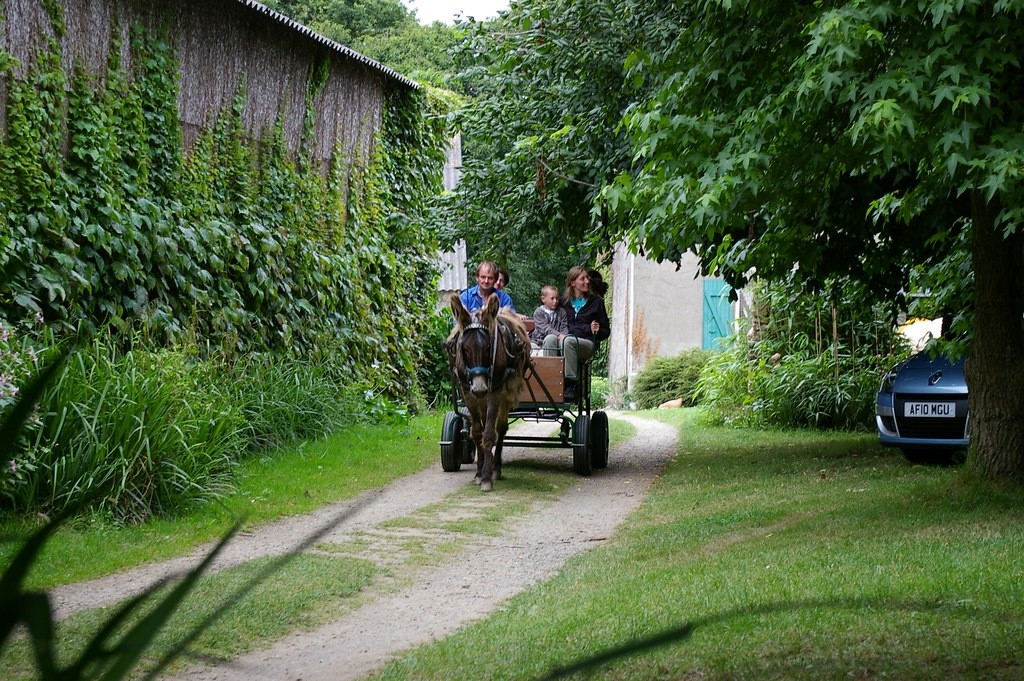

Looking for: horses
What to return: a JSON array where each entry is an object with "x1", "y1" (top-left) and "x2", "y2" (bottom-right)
[{"x1": 446, "y1": 292, "x2": 532, "y2": 491}]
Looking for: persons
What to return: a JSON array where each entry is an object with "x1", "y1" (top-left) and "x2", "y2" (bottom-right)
[
  {"x1": 453, "y1": 260, "x2": 515, "y2": 328},
  {"x1": 493, "y1": 268, "x2": 509, "y2": 290},
  {"x1": 530, "y1": 285, "x2": 568, "y2": 357},
  {"x1": 588, "y1": 269, "x2": 609, "y2": 297},
  {"x1": 560, "y1": 266, "x2": 610, "y2": 397}
]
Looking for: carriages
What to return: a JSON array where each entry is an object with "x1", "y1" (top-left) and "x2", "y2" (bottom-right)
[{"x1": 437, "y1": 294, "x2": 609, "y2": 492}]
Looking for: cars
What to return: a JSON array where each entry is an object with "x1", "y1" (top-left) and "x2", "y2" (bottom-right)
[{"x1": 875, "y1": 326, "x2": 972, "y2": 465}]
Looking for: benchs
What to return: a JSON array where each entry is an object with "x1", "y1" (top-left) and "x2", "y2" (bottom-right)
[{"x1": 524, "y1": 320, "x2": 600, "y2": 398}]
